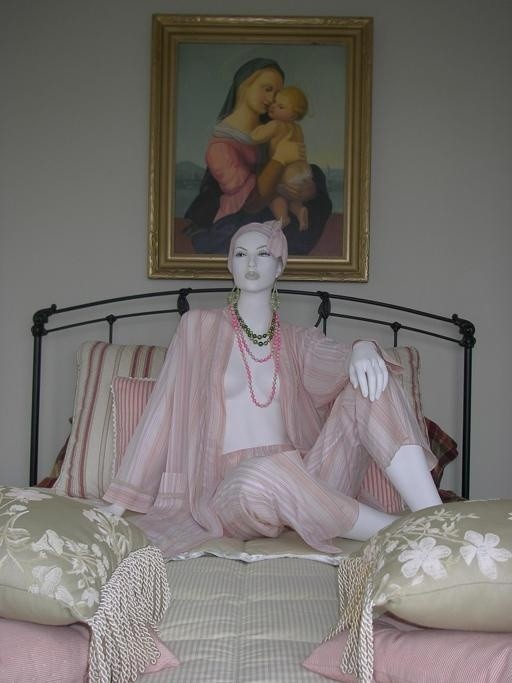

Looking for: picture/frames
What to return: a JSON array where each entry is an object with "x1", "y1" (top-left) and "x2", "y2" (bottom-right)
[{"x1": 147, "y1": 11, "x2": 374, "y2": 286}]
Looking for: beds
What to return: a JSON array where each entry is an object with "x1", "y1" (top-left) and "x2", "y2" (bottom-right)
[{"x1": 2, "y1": 285, "x2": 512, "y2": 681}]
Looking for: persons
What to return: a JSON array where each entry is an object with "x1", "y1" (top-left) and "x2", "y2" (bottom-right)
[
  {"x1": 99, "y1": 217, "x2": 446, "y2": 546},
  {"x1": 185, "y1": 52, "x2": 334, "y2": 257},
  {"x1": 250, "y1": 82, "x2": 317, "y2": 233}
]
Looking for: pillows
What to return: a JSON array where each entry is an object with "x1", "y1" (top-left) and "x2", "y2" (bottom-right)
[
  {"x1": 110, "y1": 377, "x2": 158, "y2": 481},
  {"x1": 301, "y1": 605, "x2": 512, "y2": 682},
  {"x1": 55, "y1": 341, "x2": 165, "y2": 500},
  {"x1": 322, "y1": 498, "x2": 512, "y2": 683},
  {"x1": 1, "y1": 486, "x2": 169, "y2": 682},
  {"x1": 379, "y1": 345, "x2": 430, "y2": 446},
  {"x1": 353, "y1": 456, "x2": 407, "y2": 515},
  {"x1": 0, "y1": 617, "x2": 182, "y2": 680}
]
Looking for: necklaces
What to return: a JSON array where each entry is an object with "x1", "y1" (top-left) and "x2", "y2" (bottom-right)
[
  {"x1": 230, "y1": 306, "x2": 280, "y2": 408},
  {"x1": 234, "y1": 301, "x2": 277, "y2": 347}
]
